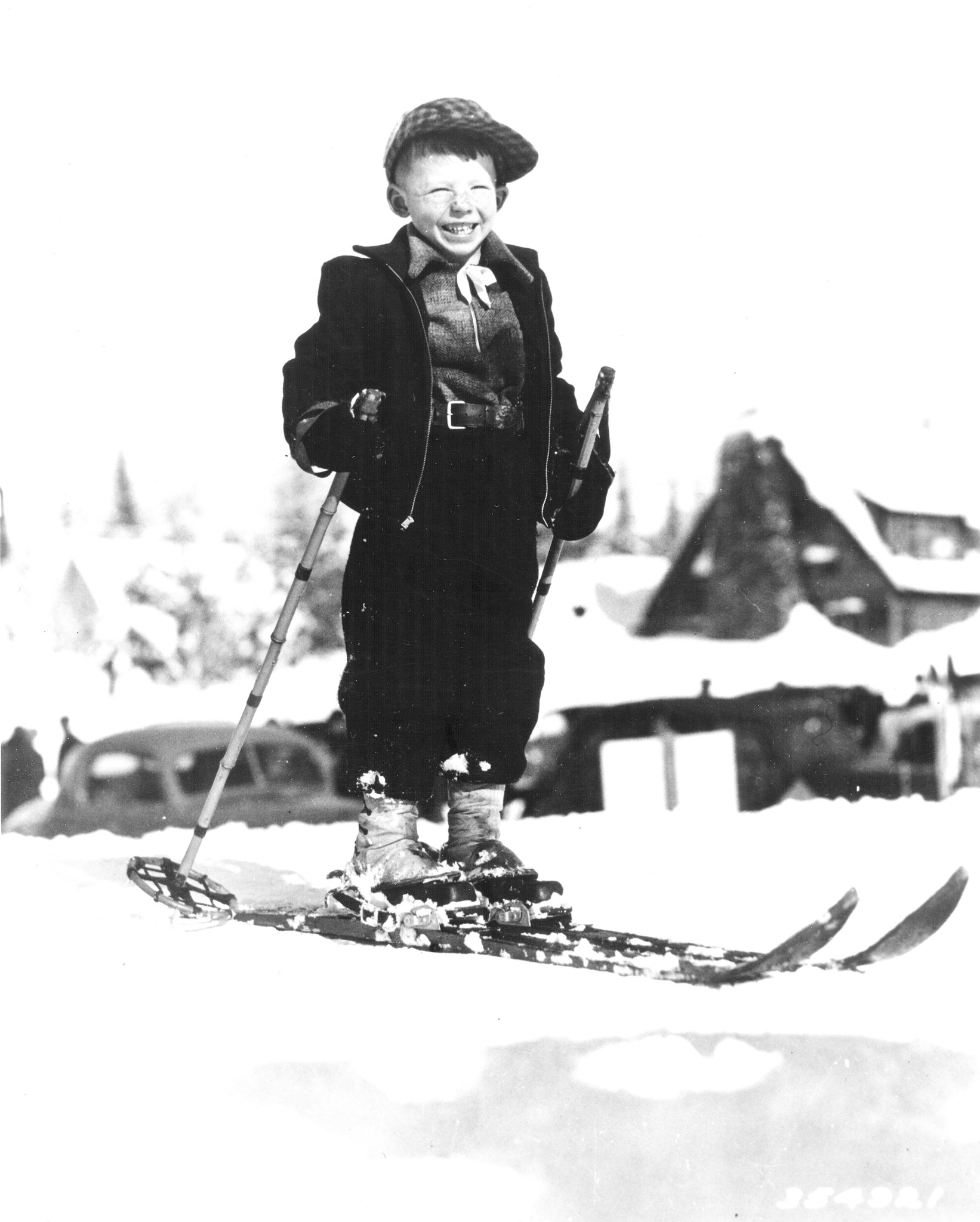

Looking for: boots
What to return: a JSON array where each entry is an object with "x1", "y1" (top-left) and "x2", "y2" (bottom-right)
[
  {"x1": 438, "y1": 774, "x2": 544, "y2": 901},
  {"x1": 341, "y1": 787, "x2": 476, "y2": 904}
]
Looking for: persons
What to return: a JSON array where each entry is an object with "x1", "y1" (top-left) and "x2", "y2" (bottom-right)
[{"x1": 283, "y1": 99, "x2": 615, "y2": 885}]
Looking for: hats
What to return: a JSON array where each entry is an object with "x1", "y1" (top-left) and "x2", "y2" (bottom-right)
[{"x1": 383, "y1": 94, "x2": 540, "y2": 188}]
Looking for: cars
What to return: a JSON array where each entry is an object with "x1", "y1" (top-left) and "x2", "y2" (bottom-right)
[{"x1": 5, "y1": 722, "x2": 364, "y2": 840}]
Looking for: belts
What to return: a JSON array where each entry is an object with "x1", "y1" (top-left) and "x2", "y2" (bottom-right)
[{"x1": 405, "y1": 401, "x2": 529, "y2": 432}]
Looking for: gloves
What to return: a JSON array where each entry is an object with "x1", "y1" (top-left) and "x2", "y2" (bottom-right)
[
  {"x1": 553, "y1": 431, "x2": 616, "y2": 541},
  {"x1": 307, "y1": 402, "x2": 398, "y2": 474}
]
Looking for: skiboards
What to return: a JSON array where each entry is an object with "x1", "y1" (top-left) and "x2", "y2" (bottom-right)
[{"x1": 232, "y1": 865, "x2": 970, "y2": 988}]
